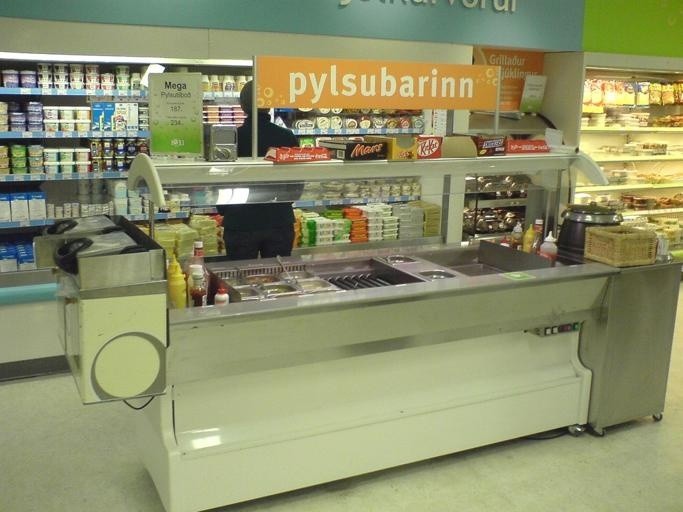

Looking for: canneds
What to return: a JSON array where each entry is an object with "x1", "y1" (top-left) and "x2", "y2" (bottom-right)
[{"x1": 90, "y1": 138, "x2": 150, "y2": 173}]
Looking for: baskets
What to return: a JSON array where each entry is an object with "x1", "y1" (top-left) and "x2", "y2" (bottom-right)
[{"x1": 585, "y1": 225, "x2": 656, "y2": 267}]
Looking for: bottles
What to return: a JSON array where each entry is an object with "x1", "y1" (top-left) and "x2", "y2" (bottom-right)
[
  {"x1": 214, "y1": 283, "x2": 230, "y2": 305},
  {"x1": 510, "y1": 216, "x2": 561, "y2": 262},
  {"x1": 202, "y1": 74, "x2": 254, "y2": 92},
  {"x1": 166, "y1": 250, "x2": 187, "y2": 310},
  {"x1": 187, "y1": 241, "x2": 211, "y2": 307}
]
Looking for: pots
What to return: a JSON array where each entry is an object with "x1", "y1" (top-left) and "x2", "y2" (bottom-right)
[{"x1": 559, "y1": 202, "x2": 624, "y2": 255}]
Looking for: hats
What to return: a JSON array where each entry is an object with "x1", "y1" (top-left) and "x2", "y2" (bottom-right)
[{"x1": 240, "y1": 80, "x2": 270, "y2": 113}]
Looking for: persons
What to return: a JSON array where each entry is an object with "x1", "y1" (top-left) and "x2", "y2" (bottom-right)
[{"x1": 215, "y1": 82, "x2": 305, "y2": 258}]
